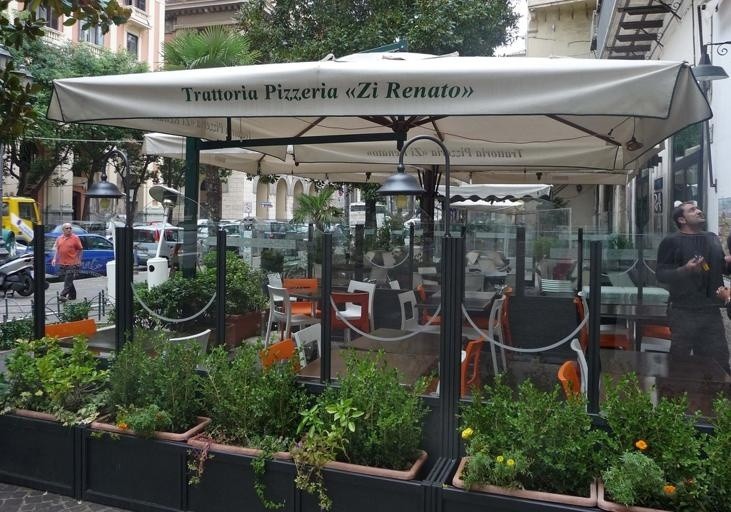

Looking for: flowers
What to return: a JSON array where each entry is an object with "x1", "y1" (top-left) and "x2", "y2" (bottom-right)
[
  {"x1": 462, "y1": 428, "x2": 473, "y2": 440},
  {"x1": 497, "y1": 455, "x2": 503, "y2": 461},
  {"x1": 636, "y1": 440, "x2": 647, "y2": 448},
  {"x1": 507, "y1": 459, "x2": 515, "y2": 465},
  {"x1": 662, "y1": 486, "x2": 675, "y2": 495}
]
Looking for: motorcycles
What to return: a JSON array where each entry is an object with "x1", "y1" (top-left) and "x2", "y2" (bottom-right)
[{"x1": 0, "y1": 249, "x2": 36, "y2": 303}]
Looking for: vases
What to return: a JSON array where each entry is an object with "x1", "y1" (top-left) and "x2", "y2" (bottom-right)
[
  {"x1": 596, "y1": 479, "x2": 655, "y2": 510},
  {"x1": 453, "y1": 456, "x2": 598, "y2": 506}
]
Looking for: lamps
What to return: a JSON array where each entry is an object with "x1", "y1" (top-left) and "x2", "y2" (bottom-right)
[
  {"x1": 81, "y1": 147, "x2": 135, "y2": 228},
  {"x1": 691, "y1": 38, "x2": 730, "y2": 82}
]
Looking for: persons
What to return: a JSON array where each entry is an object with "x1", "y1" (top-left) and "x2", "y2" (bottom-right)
[
  {"x1": 656, "y1": 201, "x2": 730, "y2": 375},
  {"x1": 716, "y1": 285, "x2": 731, "y2": 319},
  {"x1": 51, "y1": 223, "x2": 83, "y2": 300}
]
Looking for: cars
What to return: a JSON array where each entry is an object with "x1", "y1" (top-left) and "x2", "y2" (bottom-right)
[{"x1": 399, "y1": 206, "x2": 443, "y2": 230}]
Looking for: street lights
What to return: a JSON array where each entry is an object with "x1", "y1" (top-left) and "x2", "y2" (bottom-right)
[{"x1": 84, "y1": 148, "x2": 136, "y2": 359}]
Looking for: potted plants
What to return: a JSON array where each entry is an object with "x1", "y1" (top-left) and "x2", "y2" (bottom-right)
[
  {"x1": 109, "y1": 251, "x2": 269, "y2": 347},
  {"x1": 89, "y1": 326, "x2": 214, "y2": 442},
  {"x1": 0, "y1": 336, "x2": 113, "y2": 429},
  {"x1": 0, "y1": 313, "x2": 34, "y2": 348},
  {"x1": 62, "y1": 302, "x2": 90, "y2": 324},
  {"x1": 188, "y1": 337, "x2": 434, "y2": 481}
]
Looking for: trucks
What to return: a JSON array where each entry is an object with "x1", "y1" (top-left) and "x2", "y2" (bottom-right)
[{"x1": 0, "y1": 193, "x2": 42, "y2": 244}]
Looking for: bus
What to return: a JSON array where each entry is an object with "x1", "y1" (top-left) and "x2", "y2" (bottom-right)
[{"x1": 344, "y1": 201, "x2": 387, "y2": 229}]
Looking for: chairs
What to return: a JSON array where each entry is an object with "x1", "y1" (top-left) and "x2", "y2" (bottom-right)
[
  {"x1": 523, "y1": 257, "x2": 577, "y2": 297},
  {"x1": 260, "y1": 340, "x2": 488, "y2": 397},
  {"x1": 388, "y1": 252, "x2": 512, "y2": 381},
  {"x1": 266, "y1": 270, "x2": 376, "y2": 341},
  {"x1": 558, "y1": 286, "x2": 672, "y2": 413}
]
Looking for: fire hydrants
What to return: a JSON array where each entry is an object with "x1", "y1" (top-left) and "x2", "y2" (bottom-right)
[{"x1": 146, "y1": 181, "x2": 181, "y2": 291}]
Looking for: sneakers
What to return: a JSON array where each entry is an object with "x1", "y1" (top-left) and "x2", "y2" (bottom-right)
[
  {"x1": 67, "y1": 295, "x2": 77, "y2": 300},
  {"x1": 58, "y1": 296, "x2": 67, "y2": 302}
]
